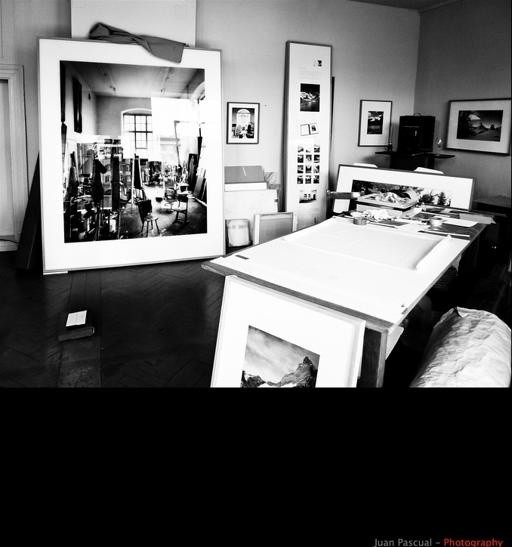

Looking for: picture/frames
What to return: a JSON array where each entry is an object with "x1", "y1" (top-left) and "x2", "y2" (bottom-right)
[
  {"x1": 282, "y1": 41, "x2": 332, "y2": 228},
  {"x1": 227, "y1": 102, "x2": 260, "y2": 145},
  {"x1": 37, "y1": 38, "x2": 225, "y2": 273},
  {"x1": 358, "y1": 99, "x2": 392, "y2": 147},
  {"x1": 211, "y1": 276, "x2": 366, "y2": 387},
  {"x1": 333, "y1": 164, "x2": 475, "y2": 214},
  {"x1": 445, "y1": 97, "x2": 512, "y2": 155}
]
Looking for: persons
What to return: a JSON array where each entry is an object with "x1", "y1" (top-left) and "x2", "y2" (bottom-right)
[
  {"x1": 246, "y1": 123, "x2": 254, "y2": 139},
  {"x1": 237, "y1": 126, "x2": 247, "y2": 139},
  {"x1": 82, "y1": 147, "x2": 111, "y2": 210}
]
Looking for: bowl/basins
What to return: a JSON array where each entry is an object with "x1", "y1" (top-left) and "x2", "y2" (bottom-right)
[{"x1": 429, "y1": 216, "x2": 443, "y2": 226}]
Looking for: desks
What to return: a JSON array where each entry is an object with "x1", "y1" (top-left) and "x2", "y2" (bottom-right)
[{"x1": 201, "y1": 212, "x2": 494, "y2": 334}]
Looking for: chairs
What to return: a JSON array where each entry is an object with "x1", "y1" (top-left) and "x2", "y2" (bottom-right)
[{"x1": 136, "y1": 200, "x2": 160, "y2": 235}]
